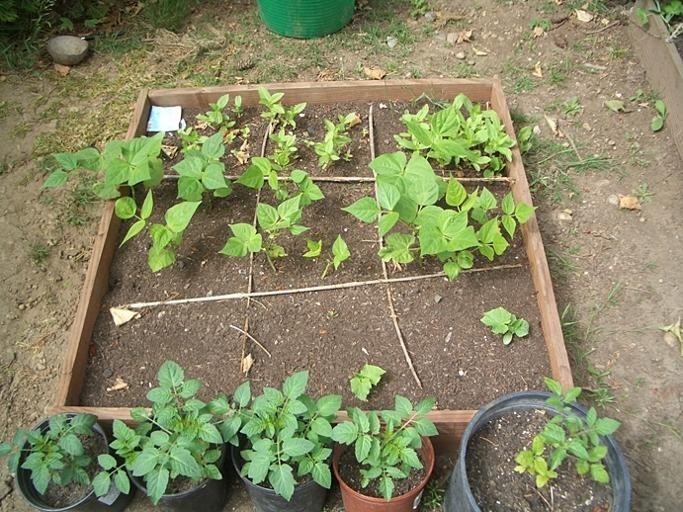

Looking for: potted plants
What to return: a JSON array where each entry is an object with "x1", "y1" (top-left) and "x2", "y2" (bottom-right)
[
  {"x1": 444, "y1": 376, "x2": 632, "y2": 512},
  {"x1": 329, "y1": 393, "x2": 439, "y2": 512},
  {"x1": 226, "y1": 371, "x2": 342, "y2": 512},
  {"x1": 93, "y1": 361, "x2": 226, "y2": 512},
  {"x1": 15, "y1": 411, "x2": 110, "y2": 511}
]
